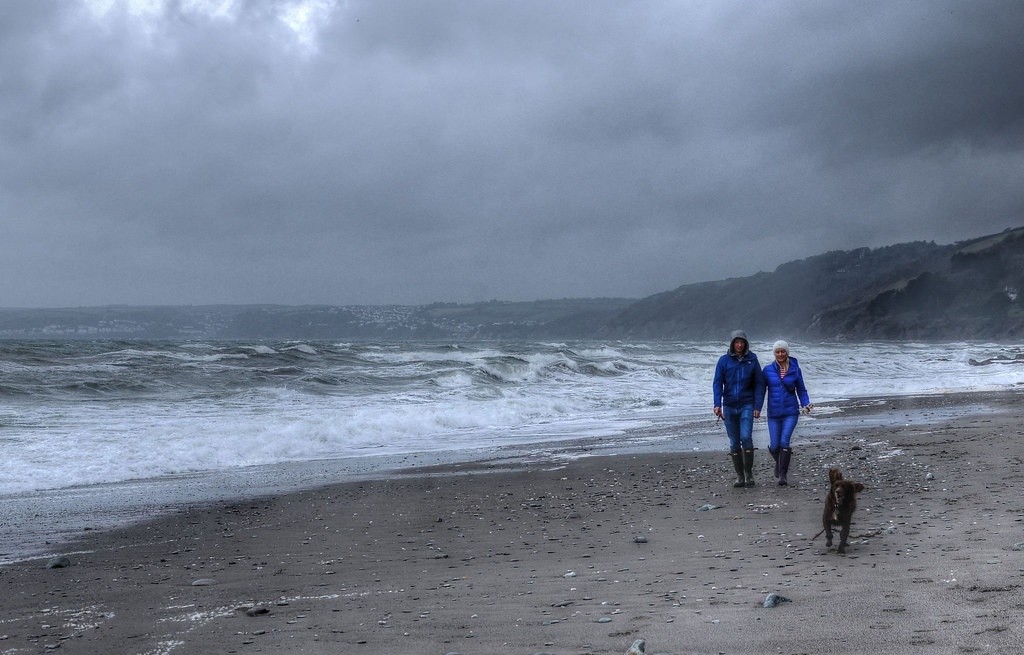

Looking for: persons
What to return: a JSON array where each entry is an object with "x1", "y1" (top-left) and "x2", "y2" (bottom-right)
[
  {"x1": 763, "y1": 340, "x2": 810, "y2": 486},
  {"x1": 713, "y1": 329, "x2": 766, "y2": 488}
]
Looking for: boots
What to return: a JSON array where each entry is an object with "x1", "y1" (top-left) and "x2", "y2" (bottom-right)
[
  {"x1": 768, "y1": 445, "x2": 779, "y2": 478},
  {"x1": 778, "y1": 447, "x2": 792, "y2": 486},
  {"x1": 727, "y1": 451, "x2": 745, "y2": 487},
  {"x1": 742, "y1": 448, "x2": 755, "y2": 487}
]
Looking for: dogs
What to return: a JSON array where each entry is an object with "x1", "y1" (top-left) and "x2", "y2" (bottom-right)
[{"x1": 822, "y1": 467, "x2": 864, "y2": 555}]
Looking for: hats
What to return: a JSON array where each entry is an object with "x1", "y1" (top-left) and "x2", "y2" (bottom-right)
[{"x1": 773, "y1": 340, "x2": 789, "y2": 356}]
